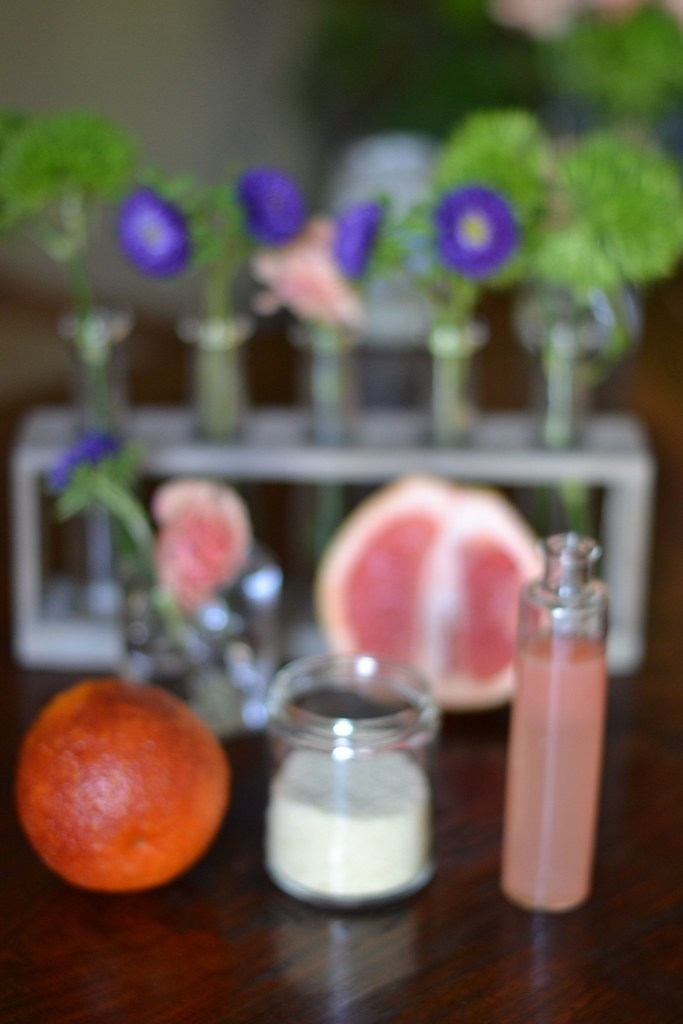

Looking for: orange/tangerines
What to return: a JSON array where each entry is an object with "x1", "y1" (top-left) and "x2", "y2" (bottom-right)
[{"x1": 14, "y1": 678, "x2": 232, "y2": 894}]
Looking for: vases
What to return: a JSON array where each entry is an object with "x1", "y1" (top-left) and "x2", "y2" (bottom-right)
[
  {"x1": 115, "y1": 546, "x2": 285, "y2": 733},
  {"x1": 174, "y1": 313, "x2": 254, "y2": 438},
  {"x1": 53, "y1": 310, "x2": 140, "y2": 619},
  {"x1": 288, "y1": 324, "x2": 367, "y2": 587},
  {"x1": 417, "y1": 321, "x2": 496, "y2": 447},
  {"x1": 530, "y1": 328, "x2": 604, "y2": 540}
]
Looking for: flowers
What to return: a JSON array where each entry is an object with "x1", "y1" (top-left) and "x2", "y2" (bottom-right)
[{"x1": 0, "y1": 102, "x2": 683, "y2": 699}]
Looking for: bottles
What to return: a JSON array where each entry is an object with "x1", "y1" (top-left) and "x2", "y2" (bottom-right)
[
  {"x1": 500, "y1": 533, "x2": 603, "y2": 912},
  {"x1": 263, "y1": 656, "x2": 439, "y2": 907}
]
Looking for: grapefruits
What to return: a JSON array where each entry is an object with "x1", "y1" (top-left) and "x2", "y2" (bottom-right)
[{"x1": 314, "y1": 480, "x2": 550, "y2": 712}]
